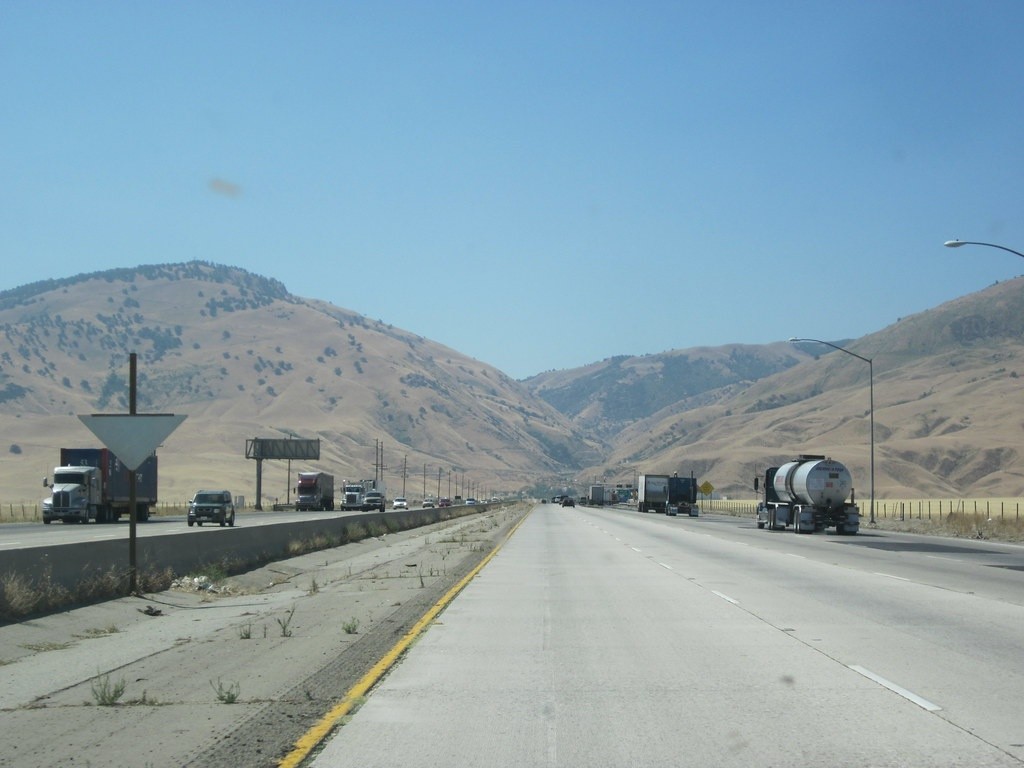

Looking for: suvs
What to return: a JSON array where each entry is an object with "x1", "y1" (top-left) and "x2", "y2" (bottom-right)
[
  {"x1": 550, "y1": 494, "x2": 575, "y2": 507},
  {"x1": 362, "y1": 491, "x2": 385, "y2": 512},
  {"x1": 187, "y1": 490, "x2": 235, "y2": 527},
  {"x1": 579, "y1": 497, "x2": 586, "y2": 504}
]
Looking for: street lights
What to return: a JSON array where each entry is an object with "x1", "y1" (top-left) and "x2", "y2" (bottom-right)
[
  {"x1": 403, "y1": 455, "x2": 408, "y2": 497},
  {"x1": 374, "y1": 439, "x2": 385, "y2": 481},
  {"x1": 423, "y1": 465, "x2": 496, "y2": 500},
  {"x1": 287, "y1": 432, "x2": 293, "y2": 504}
]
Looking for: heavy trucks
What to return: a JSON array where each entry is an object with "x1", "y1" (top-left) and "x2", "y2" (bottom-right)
[
  {"x1": 42, "y1": 448, "x2": 158, "y2": 524},
  {"x1": 789, "y1": 337, "x2": 875, "y2": 523},
  {"x1": 340, "y1": 479, "x2": 385, "y2": 511},
  {"x1": 588, "y1": 486, "x2": 604, "y2": 505},
  {"x1": 663, "y1": 470, "x2": 699, "y2": 517},
  {"x1": 294, "y1": 471, "x2": 334, "y2": 511},
  {"x1": 636, "y1": 474, "x2": 670, "y2": 512},
  {"x1": 754, "y1": 455, "x2": 860, "y2": 536}
]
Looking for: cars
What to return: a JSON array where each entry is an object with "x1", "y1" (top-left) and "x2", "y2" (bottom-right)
[
  {"x1": 423, "y1": 499, "x2": 434, "y2": 508},
  {"x1": 439, "y1": 499, "x2": 452, "y2": 507},
  {"x1": 466, "y1": 498, "x2": 475, "y2": 505},
  {"x1": 481, "y1": 500, "x2": 487, "y2": 503},
  {"x1": 392, "y1": 497, "x2": 408, "y2": 510}
]
